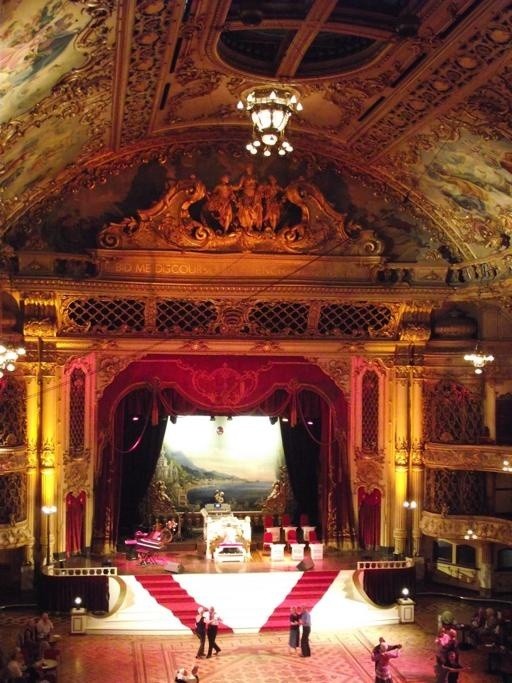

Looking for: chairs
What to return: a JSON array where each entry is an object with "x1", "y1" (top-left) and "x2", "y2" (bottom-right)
[
  {"x1": 454, "y1": 606, "x2": 512, "y2": 683},
  {"x1": 2, "y1": 617, "x2": 63, "y2": 683},
  {"x1": 262, "y1": 513, "x2": 325, "y2": 560}
]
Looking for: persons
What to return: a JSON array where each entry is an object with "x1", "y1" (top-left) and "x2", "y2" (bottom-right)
[
  {"x1": 24, "y1": 617, "x2": 40, "y2": 661},
  {"x1": 197, "y1": 173, "x2": 240, "y2": 232},
  {"x1": 293, "y1": 604, "x2": 312, "y2": 657},
  {"x1": 35, "y1": 612, "x2": 54, "y2": 641},
  {"x1": 430, "y1": 649, "x2": 472, "y2": 682},
  {"x1": 175, "y1": 664, "x2": 197, "y2": 682},
  {"x1": 192, "y1": 606, "x2": 208, "y2": 658},
  {"x1": 205, "y1": 606, "x2": 222, "y2": 659},
  {"x1": 228, "y1": 161, "x2": 262, "y2": 232},
  {"x1": 459, "y1": 606, "x2": 511, "y2": 682},
  {"x1": 369, "y1": 636, "x2": 402, "y2": 673},
  {"x1": 436, "y1": 627, "x2": 460, "y2": 663},
  {"x1": 288, "y1": 606, "x2": 300, "y2": 654},
  {"x1": 432, "y1": 626, "x2": 448, "y2": 656},
  {"x1": 8, "y1": 647, "x2": 43, "y2": 683},
  {"x1": 431, "y1": 655, "x2": 472, "y2": 682},
  {"x1": 257, "y1": 173, "x2": 290, "y2": 232},
  {"x1": 189, "y1": 662, "x2": 200, "y2": 682},
  {"x1": 369, "y1": 642, "x2": 402, "y2": 682}
]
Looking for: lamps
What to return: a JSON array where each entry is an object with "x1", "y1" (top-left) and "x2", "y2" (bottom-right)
[
  {"x1": 464, "y1": 341, "x2": 494, "y2": 374},
  {"x1": 236, "y1": 81, "x2": 304, "y2": 157}
]
